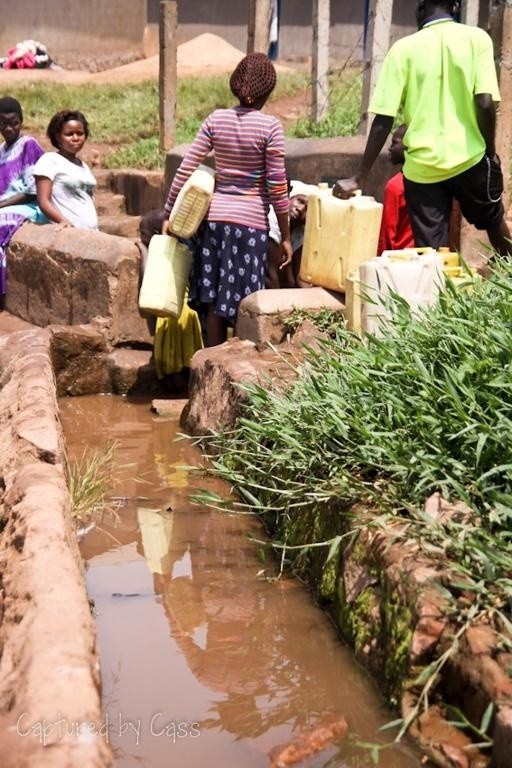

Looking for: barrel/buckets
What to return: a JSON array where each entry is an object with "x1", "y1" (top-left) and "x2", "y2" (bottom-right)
[
  {"x1": 359, "y1": 245, "x2": 432, "y2": 335},
  {"x1": 298, "y1": 181, "x2": 385, "y2": 291},
  {"x1": 344, "y1": 270, "x2": 361, "y2": 335},
  {"x1": 437, "y1": 246, "x2": 460, "y2": 277},
  {"x1": 168, "y1": 164, "x2": 216, "y2": 238},
  {"x1": 407, "y1": 247, "x2": 442, "y2": 268},
  {"x1": 137, "y1": 234, "x2": 196, "y2": 320}
]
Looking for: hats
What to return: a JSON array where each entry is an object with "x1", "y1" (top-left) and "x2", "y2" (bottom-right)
[{"x1": 231, "y1": 54, "x2": 276, "y2": 106}]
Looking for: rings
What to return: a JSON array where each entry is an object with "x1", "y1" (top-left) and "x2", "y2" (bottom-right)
[{"x1": 337, "y1": 193, "x2": 342, "y2": 197}]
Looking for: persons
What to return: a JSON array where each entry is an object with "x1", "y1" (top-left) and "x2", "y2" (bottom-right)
[
  {"x1": 136, "y1": 209, "x2": 214, "y2": 398},
  {"x1": 0, "y1": 96, "x2": 44, "y2": 304},
  {"x1": 31, "y1": 108, "x2": 99, "y2": 232},
  {"x1": 375, "y1": 121, "x2": 416, "y2": 257},
  {"x1": 267, "y1": 183, "x2": 324, "y2": 289},
  {"x1": 331, "y1": 0, "x2": 512, "y2": 263},
  {"x1": 163, "y1": 53, "x2": 293, "y2": 347}
]
